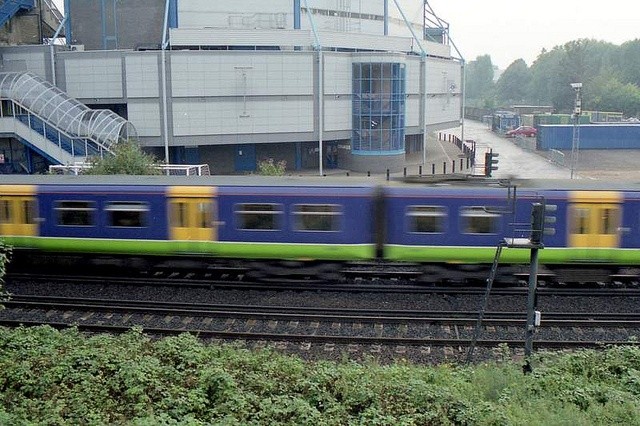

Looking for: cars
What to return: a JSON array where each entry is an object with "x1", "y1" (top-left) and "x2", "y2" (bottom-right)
[{"x1": 505, "y1": 126, "x2": 537, "y2": 137}]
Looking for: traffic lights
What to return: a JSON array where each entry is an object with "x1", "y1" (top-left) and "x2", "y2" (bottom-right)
[
  {"x1": 485, "y1": 149, "x2": 499, "y2": 176},
  {"x1": 531, "y1": 201, "x2": 557, "y2": 243}
]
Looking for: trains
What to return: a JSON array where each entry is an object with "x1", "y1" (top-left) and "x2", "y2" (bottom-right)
[{"x1": 0, "y1": 184, "x2": 639, "y2": 284}]
